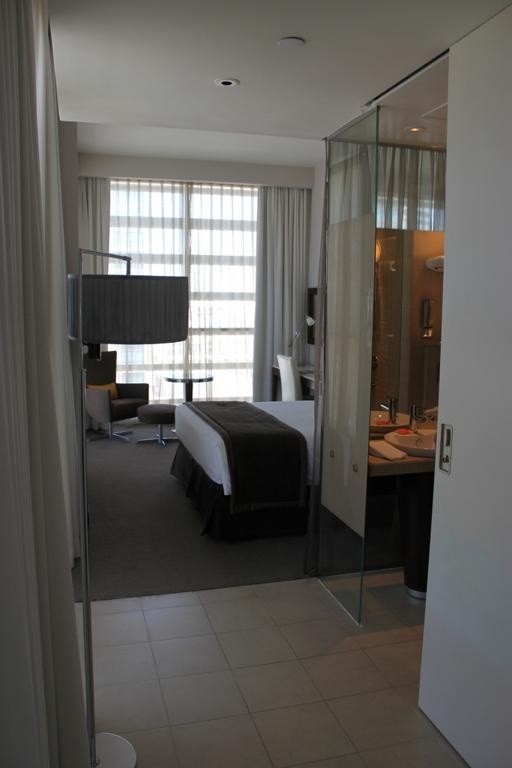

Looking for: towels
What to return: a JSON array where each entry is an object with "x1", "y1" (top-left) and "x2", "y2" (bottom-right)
[{"x1": 368, "y1": 440, "x2": 408, "y2": 461}]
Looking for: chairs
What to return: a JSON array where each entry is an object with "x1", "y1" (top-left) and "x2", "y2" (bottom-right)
[
  {"x1": 276, "y1": 353, "x2": 302, "y2": 401},
  {"x1": 82, "y1": 350, "x2": 150, "y2": 444}
]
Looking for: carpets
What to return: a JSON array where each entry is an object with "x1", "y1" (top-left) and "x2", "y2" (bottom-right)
[{"x1": 85, "y1": 426, "x2": 309, "y2": 602}]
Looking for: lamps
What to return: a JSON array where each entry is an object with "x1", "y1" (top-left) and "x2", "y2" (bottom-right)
[
  {"x1": 69, "y1": 244, "x2": 190, "y2": 768},
  {"x1": 287, "y1": 313, "x2": 315, "y2": 368}
]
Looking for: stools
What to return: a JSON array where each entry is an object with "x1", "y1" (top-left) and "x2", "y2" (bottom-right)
[{"x1": 137, "y1": 403, "x2": 180, "y2": 449}]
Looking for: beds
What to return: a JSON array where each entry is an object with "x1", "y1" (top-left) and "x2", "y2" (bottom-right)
[{"x1": 175, "y1": 400, "x2": 315, "y2": 544}]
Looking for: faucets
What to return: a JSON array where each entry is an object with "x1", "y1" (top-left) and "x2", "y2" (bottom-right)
[
  {"x1": 408, "y1": 403, "x2": 425, "y2": 432},
  {"x1": 379, "y1": 396, "x2": 398, "y2": 424}
]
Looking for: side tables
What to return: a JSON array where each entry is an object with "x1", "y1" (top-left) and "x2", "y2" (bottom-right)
[{"x1": 164, "y1": 374, "x2": 214, "y2": 433}]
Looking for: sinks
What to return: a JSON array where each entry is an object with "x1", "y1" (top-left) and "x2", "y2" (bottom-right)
[
  {"x1": 369, "y1": 409, "x2": 416, "y2": 433},
  {"x1": 383, "y1": 428, "x2": 437, "y2": 458}
]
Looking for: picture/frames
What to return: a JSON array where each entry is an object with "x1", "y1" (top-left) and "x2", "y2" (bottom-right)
[{"x1": 306, "y1": 288, "x2": 319, "y2": 345}]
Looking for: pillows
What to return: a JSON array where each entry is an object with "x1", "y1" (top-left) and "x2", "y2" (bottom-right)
[{"x1": 86, "y1": 382, "x2": 119, "y2": 401}]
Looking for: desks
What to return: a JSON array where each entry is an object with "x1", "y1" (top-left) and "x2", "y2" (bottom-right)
[{"x1": 271, "y1": 364, "x2": 321, "y2": 402}]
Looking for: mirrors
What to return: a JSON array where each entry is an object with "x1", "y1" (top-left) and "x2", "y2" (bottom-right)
[{"x1": 368, "y1": 228, "x2": 415, "y2": 414}]
